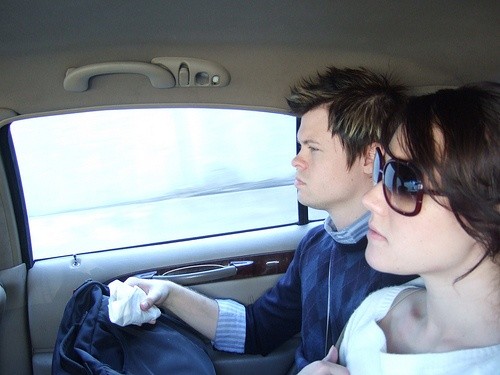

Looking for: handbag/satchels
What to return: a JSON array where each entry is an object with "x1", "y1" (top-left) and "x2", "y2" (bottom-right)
[{"x1": 49, "y1": 278, "x2": 217, "y2": 375}]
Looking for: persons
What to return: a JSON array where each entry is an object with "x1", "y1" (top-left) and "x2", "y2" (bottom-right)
[
  {"x1": 296, "y1": 82, "x2": 498, "y2": 375},
  {"x1": 125, "y1": 65, "x2": 412, "y2": 375}
]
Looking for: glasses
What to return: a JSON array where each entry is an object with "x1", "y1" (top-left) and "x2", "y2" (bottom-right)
[{"x1": 372, "y1": 147, "x2": 450, "y2": 216}]
